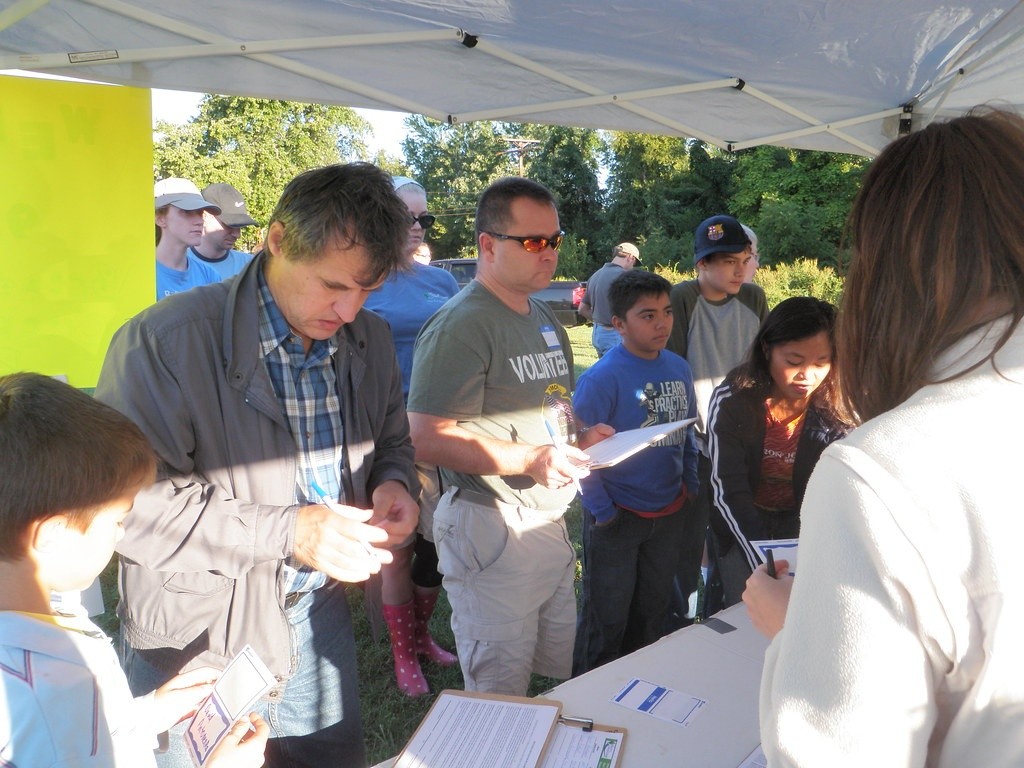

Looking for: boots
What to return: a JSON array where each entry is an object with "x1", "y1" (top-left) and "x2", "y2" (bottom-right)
[
  {"x1": 382, "y1": 595, "x2": 430, "y2": 697},
  {"x1": 413, "y1": 590, "x2": 457, "y2": 668}
]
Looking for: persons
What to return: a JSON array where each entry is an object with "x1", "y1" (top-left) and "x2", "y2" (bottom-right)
[
  {"x1": 577, "y1": 242, "x2": 645, "y2": 359},
  {"x1": 570, "y1": 269, "x2": 700, "y2": 670},
  {"x1": 154, "y1": 178, "x2": 222, "y2": 302},
  {"x1": 362, "y1": 174, "x2": 460, "y2": 696},
  {"x1": 93, "y1": 162, "x2": 423, "y2": 768},
  {"x1": 405, "y1": 177, "x2": 616, "y2": 699},
  {"x1": 667, "y1": 214, "x2": 769, "y2": 617},
  {"x1": 187, "y1": 182, "x2": 256, "y2": 283},
  {"x1": 744, "y1": 104, "x2": 1024, "y2": 768},
  {"x1": 707, "y1": 295, "x2": 848, "y2": 605},
  {"x1": 731, "y1": 224, "x2": 759, "y2": 283},
  {"x1": 0, "y1": 371, "x2": 270, "y2": 768}
]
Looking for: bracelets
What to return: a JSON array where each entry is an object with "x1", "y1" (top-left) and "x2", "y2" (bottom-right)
[{"x1": 576, "y1": 427, "x2": 586, "y2": 433}]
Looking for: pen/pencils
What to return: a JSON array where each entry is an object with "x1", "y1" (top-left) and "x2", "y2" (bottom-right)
[
  {"x1": 546, "y1": 420, "x2": 583, "y2": 494},
  {"x1": 312, "y1": 480, "x2": 382, "y2": 572},
  {"x1": 766, "y1": 548, "x2": 778, "y2": 580}
]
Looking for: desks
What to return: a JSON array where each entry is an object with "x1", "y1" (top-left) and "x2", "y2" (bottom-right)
[{"x1": 371, "y1": 601, "x2": 771, "y2": 768}]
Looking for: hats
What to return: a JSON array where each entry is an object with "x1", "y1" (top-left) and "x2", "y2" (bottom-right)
[
  {"x1": 200, "y1": 183, "x2": 259, "y2": 228},
  {"x1": 611, "y1": 243, "x2": 643, "y2": 267},
  {"x1": 694, "y1": 215, "x2": 752, "y2": 266},
  {"x1": 154, "y1": 178, "x2": 222, "y2": 216}
]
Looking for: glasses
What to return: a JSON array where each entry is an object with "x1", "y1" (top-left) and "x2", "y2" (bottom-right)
[
  {"x1": 477, "y1": 231, "x2": 565, "y2": 253},
  {"x1": 406, "y1": 214, "x2": 436, "y2": 229}
]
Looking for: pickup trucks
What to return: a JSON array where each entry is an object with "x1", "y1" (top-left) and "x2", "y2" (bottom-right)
[{"x1": 428, "y1": 258, "x2": 588, "y2": 327}]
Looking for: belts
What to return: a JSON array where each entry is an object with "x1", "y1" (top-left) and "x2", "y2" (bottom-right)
[{"x1": 284, "y1": 592, "x2": 312, "y2": 613}]
